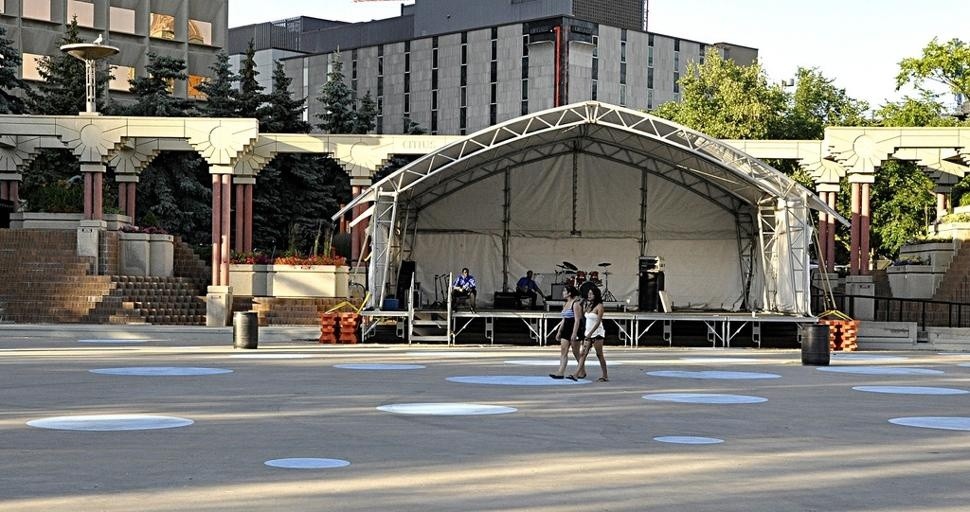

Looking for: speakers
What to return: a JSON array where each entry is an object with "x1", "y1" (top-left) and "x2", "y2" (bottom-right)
[
  {"x1": 639, "y1": 271, "x2": 664, "y2": 313},
  {"x1": 397, "y1": 260, "x2": 415, "y2": 309}
]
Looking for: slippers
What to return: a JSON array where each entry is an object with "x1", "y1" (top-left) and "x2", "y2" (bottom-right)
[
  {"x1": 566, "y1": 374, "x2": 577, "y2": 382},
  {"x1": 598, "y1": 376, "x2": 608, "y2": 382}
]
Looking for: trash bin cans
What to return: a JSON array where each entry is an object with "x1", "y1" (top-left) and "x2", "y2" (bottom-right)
[
  {"x1": 801, "y1": 323, "x2": 830, "y2": 366},
  {"x1": 233, "y1": 311, "x2": 258, "y2": 349},
  {"x1": 205, "y1": 293, "x2": 228, "y2": 327}
]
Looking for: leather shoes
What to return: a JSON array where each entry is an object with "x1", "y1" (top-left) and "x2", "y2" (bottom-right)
[
  {"x1": 577, "y1": 373, "x2": 586, "y2": 379},
  {"x1": 549, "y1": 374, "x2": 564, "y2": 379}
]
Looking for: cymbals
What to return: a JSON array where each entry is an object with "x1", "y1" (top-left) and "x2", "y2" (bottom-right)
[
  {"x1": 562, "y1": 262, "x2": 578, "y2": 271},
  {"x1": 598, "y1": 263, "x2": 611, "y2": 266},
  {"x1": 556, "y1": 264, "x2": 569, "y2": 269},
  {"x1": 602, "y1": 271, "x2": 612, "y2": 274}
]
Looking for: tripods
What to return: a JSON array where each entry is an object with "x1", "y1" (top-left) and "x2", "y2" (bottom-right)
[{"x1": 602, "y1": 274, "x2": 617, "y2": 301}]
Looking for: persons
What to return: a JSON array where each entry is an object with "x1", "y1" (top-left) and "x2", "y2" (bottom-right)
[
  {"x1": 515, "y1": 270, "x2": 546, "y2": 310},
  {"x1": 451, "y1": 267, "x2": 477, "y2": 314},
  {"x1": 549, "y1": 285, "x2": 609, "y2": 382}
]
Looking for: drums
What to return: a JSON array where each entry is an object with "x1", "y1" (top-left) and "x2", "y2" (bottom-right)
[
  {"x1": 589, "y1": 271, "x2": 598, "y2": 281},
  {"x1": 576, "y1": 271, "x2": 586, "y2": 281}
]
